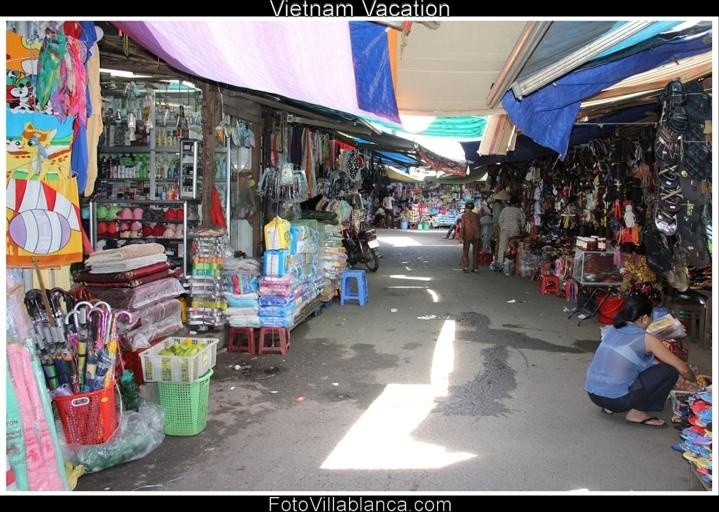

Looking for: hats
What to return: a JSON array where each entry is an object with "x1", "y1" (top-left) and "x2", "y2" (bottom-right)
[
  {"x1": 493, "y1": 189, "x2": 511, "y2": 201},
  {"x1": 643, "y1": 80, "x2": 712, "y2": 293}
]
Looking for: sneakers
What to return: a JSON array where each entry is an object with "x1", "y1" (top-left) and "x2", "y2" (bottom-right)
[{"x1": 462, "y1": 267, "x2": 478, "y2": 272}]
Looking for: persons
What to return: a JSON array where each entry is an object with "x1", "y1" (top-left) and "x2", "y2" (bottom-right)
[
  {"x1": 455, "y1": 188, "x2": 527, "y2": 275},
  {"x1": 381, "y1": 192, "x2": 396, "y2": 230},
  {"x1": 582, "y1": 293, "x2": 699, "y2": 427},
  {"x1": 440, "y1": 212, "x2": 464, "y2": 239},
  {"x1": 607, "y1": 241, "x2": 665, "y2": 298}
]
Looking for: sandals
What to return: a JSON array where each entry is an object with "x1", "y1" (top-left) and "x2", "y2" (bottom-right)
[{"x1": 670, "y1": 384, "x2": 712, "y2": 484}]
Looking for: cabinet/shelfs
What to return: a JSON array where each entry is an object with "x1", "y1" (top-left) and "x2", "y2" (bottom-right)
[{"x1": 80, "y1": 140, "x2": 230, "y2": 280}]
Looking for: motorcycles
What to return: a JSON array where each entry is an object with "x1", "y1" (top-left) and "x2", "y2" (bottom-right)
[{"x1": 340, "y1": 221, "x2": 383, "y2": 273}]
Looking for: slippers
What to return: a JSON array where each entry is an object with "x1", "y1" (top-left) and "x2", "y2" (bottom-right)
[{"x1": 601, "y1": 408, "x2": 668, "y2": 429}]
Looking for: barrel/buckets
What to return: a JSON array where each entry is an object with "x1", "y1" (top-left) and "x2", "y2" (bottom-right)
[{"x1": 597, "y1": 291, "x2": 624, "y2": 323}]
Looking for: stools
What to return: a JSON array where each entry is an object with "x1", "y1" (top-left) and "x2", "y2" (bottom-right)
[
  {"x1": 226, "y1": 327, "x2": 291, "y2": 356},
  {"x1": 340, "y1": 269, "x2": 369, "y2": 306},
  {"x1": 539, "y1": 275, "x2": 561, "y2": 296}
]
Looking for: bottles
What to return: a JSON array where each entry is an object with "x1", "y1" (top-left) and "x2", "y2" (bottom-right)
[{"x1": 93, "y1": 108, "x2": 180, "y2": 201}]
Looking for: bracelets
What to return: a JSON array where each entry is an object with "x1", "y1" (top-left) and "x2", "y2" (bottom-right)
[{"x1": 681, "y1": 370, "x2": 696, "y2": 383}]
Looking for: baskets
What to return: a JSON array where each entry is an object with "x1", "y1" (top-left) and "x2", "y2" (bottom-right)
[
  {"x1": 138, "y1": 335, "x2": 220, "y2": 436},
  {"x1": 52, "y1": 378, "x2": 119, "y2": 445}
]
